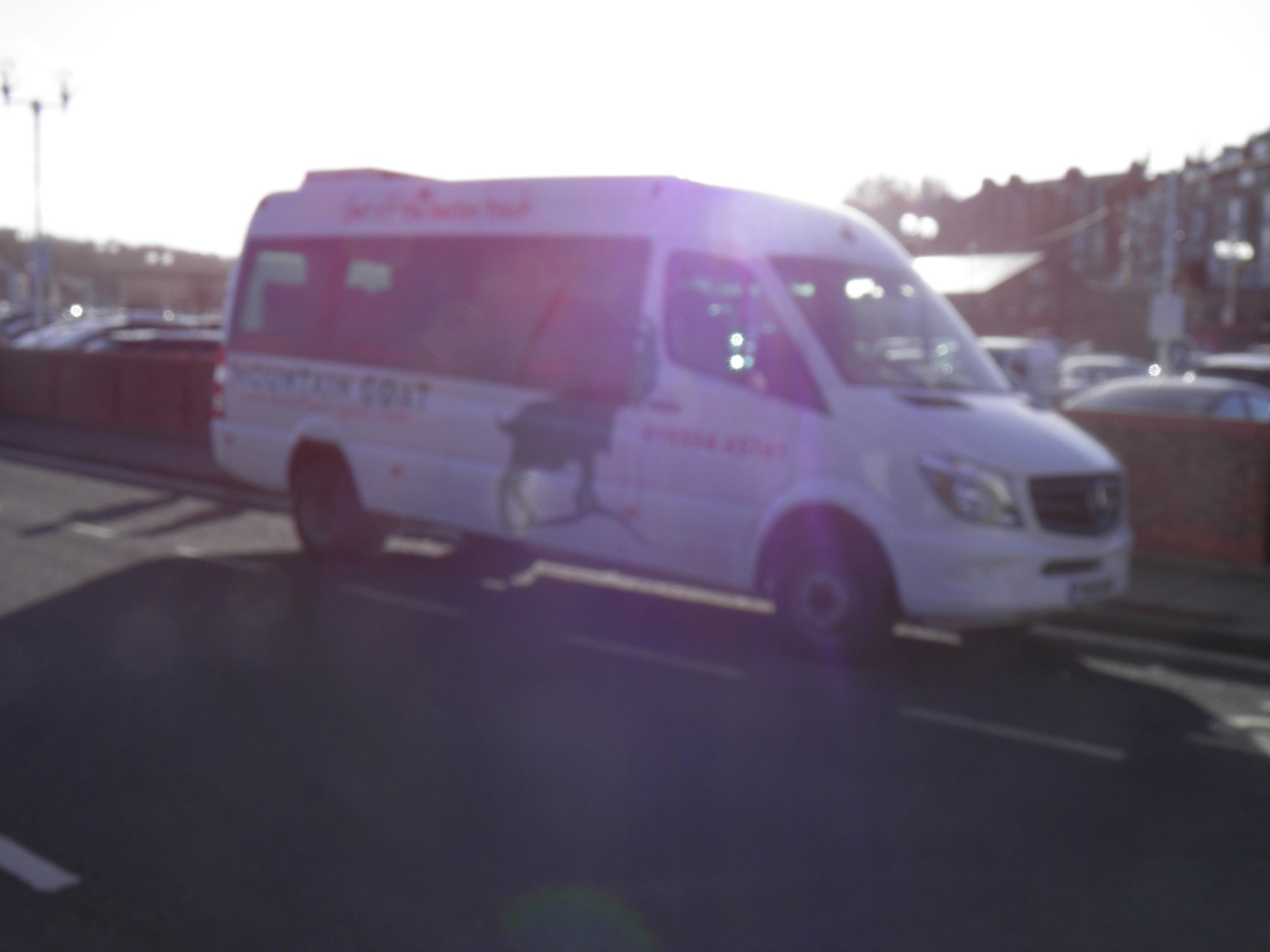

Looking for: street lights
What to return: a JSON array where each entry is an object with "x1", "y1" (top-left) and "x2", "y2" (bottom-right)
[{"x1": 0, "y1": 78, "x2": 74, "y2": 327}]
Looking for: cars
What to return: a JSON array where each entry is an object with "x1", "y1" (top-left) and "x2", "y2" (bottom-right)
[
  {"x1": 2, "y1": 302, "x2": 222, "y2": 350},
  {"x1": 889, "y1": 331, "x2": 1270, "y2": 428}
]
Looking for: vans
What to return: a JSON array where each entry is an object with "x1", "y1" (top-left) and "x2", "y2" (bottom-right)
[{"x1": 206, "y1": 165, "x2": 1121, "y2": 643}]
